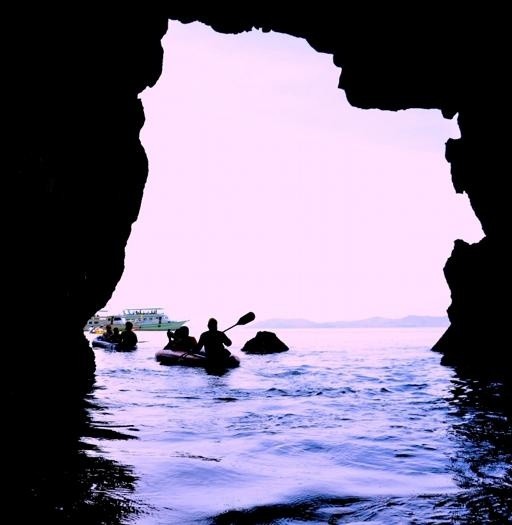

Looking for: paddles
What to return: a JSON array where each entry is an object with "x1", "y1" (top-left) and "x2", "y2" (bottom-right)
[{"x1": 224, "y1": 312, "x2": 255, "y2": 333}]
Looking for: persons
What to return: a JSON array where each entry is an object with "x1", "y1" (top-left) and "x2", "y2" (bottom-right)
[{"x1": 103, "y1": 317, "x2": 232, "y2": 355}]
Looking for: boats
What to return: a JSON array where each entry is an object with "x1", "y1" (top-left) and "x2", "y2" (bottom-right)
[
  {"x1": 91, "y1": 327, "x2": 107, "y2": 334},
  {"x1": 153, "y1": 350, "x2": 240, "y2": 369},
  {"x1": 92, "y1": 335, "x2": 138, "y2": 352},
  {"x1": 84, "y1": 307, "x2": 191, "y2": 331}
]
